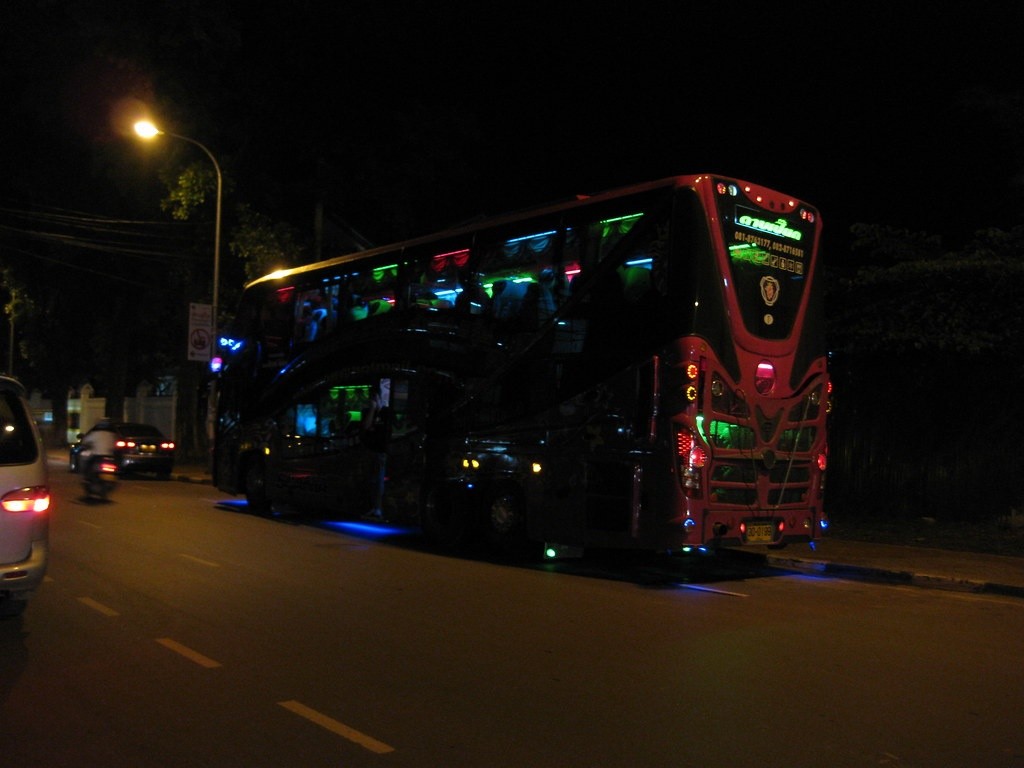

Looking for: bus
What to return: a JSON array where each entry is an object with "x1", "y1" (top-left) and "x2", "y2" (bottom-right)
[{"x1": 213, "y1": 174, "x2": 831, "y2": 566}]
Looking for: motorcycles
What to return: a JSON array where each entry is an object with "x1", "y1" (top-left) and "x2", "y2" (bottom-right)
[{"x1": 77, "y1": 434, "x2": 121, "y2": 498}]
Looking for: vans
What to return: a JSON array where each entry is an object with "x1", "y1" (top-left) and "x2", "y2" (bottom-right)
[{"x1": 0, "y1": 374, "x2": 49, "y2": 591}]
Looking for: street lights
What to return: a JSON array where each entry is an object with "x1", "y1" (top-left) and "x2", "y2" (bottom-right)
[{"x1": 132, "y1": 113, "x2": 221, "y2": 474}]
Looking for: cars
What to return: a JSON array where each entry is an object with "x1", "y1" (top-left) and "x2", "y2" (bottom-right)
[{"x1": 70, "y1": 421, "x2": 177, "y2": 479}]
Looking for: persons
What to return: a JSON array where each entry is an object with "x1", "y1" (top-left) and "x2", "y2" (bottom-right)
[
  {"x1": 453, "y1": 267, "x2": 493, "y2": 303},
  {"x1": 359, "y1": 387, "x2": 405, "y2": 435},
  {"x1": 81, "y1": 415, "x2": 117, "y2": 459}
]
